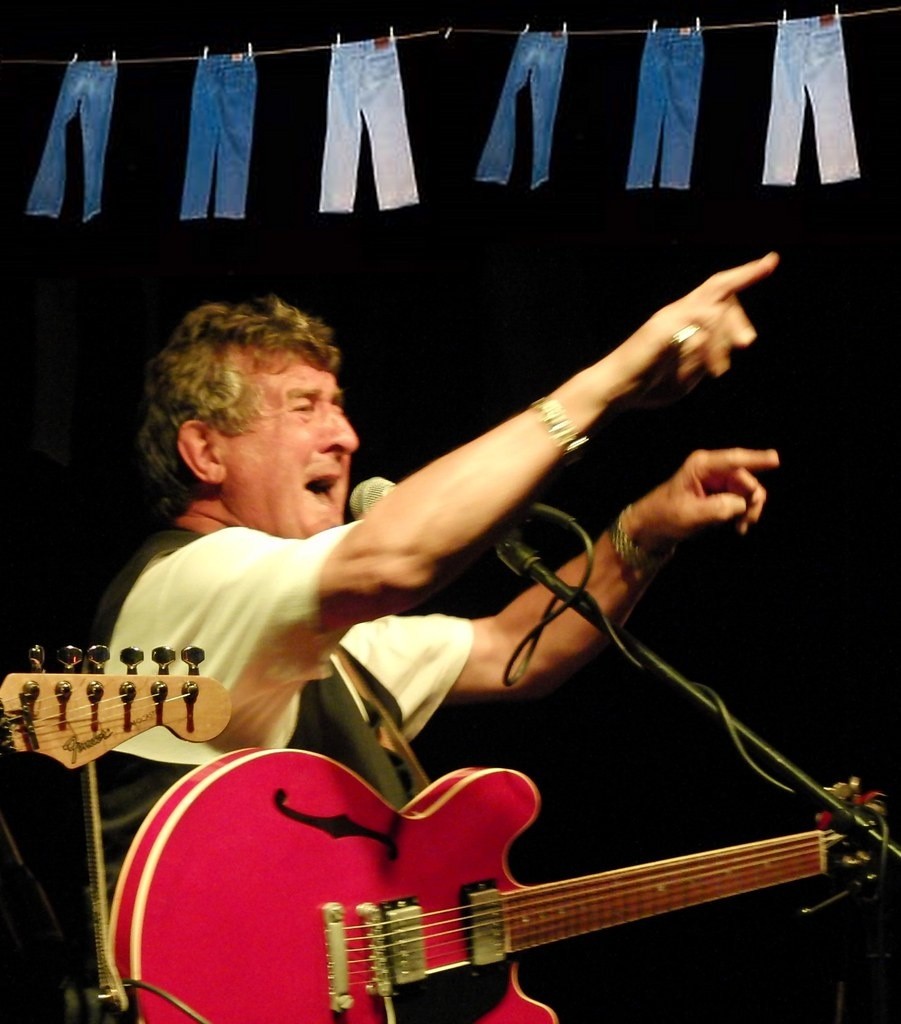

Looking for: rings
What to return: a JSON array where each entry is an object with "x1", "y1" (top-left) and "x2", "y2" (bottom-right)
[{"x1": 666, "y1": 323, "x2": 701, "y2": 351}]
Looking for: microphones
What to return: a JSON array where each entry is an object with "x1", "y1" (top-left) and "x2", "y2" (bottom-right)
[{"x1": 348, "y1": 479, "x2": 395, "y2": 521}]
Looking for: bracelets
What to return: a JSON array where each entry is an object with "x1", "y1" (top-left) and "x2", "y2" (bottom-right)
[
  {"x1": 611, "y1": 502, "x2": 680, "y2": 572},
  {"x1": 527, "y1": 396, "x2": 589, "y2": 462}
]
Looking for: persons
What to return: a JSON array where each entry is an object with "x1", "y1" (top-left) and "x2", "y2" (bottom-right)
[{"x1": 80, "y1": 253, "x2": 782, "y2": 905}]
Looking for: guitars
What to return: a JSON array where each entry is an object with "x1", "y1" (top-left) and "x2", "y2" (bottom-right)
[
  {"x1": 0, "y1": 644, "x2": 234, "y2": 769},
  {"x1": 104, "y1": 744, "x2": 885, "y2": 1024}
]
